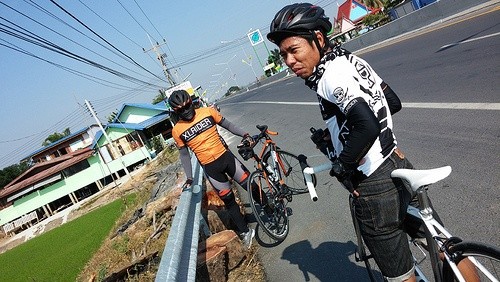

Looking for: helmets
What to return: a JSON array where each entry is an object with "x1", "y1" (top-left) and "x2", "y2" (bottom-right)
[
  {"x1": 266, "y1": 2, "x2": 332, "y2": 47},
  {"x1": 168, "y1": 90, "x2": 192, "y2": 111}
]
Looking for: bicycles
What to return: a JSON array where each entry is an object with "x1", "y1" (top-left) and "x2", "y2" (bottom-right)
[
  {"x1": 309, "y1": 128, "x2": 500, "y2": 282},
  {"x1": 237, "y1": 125, "x2": 317, "y2": 242}
]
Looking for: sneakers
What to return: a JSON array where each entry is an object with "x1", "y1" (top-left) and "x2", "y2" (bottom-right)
[
  {"x1": 267, "y1": 206, "x2": 294, "y2": 219},
  {"x1": 240, "y1": 228, "x2": 255, "y2": 249}
]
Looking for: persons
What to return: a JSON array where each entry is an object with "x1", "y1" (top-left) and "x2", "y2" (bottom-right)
[
  {"x1": 267, "y1": 3, "x2": 481, "y2": 282},
  {"x1": 168, "y1": 90, "x2": 283, "y2": 250}
]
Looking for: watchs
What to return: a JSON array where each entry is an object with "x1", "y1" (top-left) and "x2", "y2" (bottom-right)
[{"x1": 333, "y1": 160, "x2": 345, "y2": 174}]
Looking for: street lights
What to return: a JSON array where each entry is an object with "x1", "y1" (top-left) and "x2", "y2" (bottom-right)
[
  {"x1": 209, "y1": 67, "x2": 229, "y2": 89},
  {"x1": 214, "y1": 54, "x2": 239, "y2": 87},
  {"x1": 220, "y1": 28, "x2": 258, "y2": 77}
]
